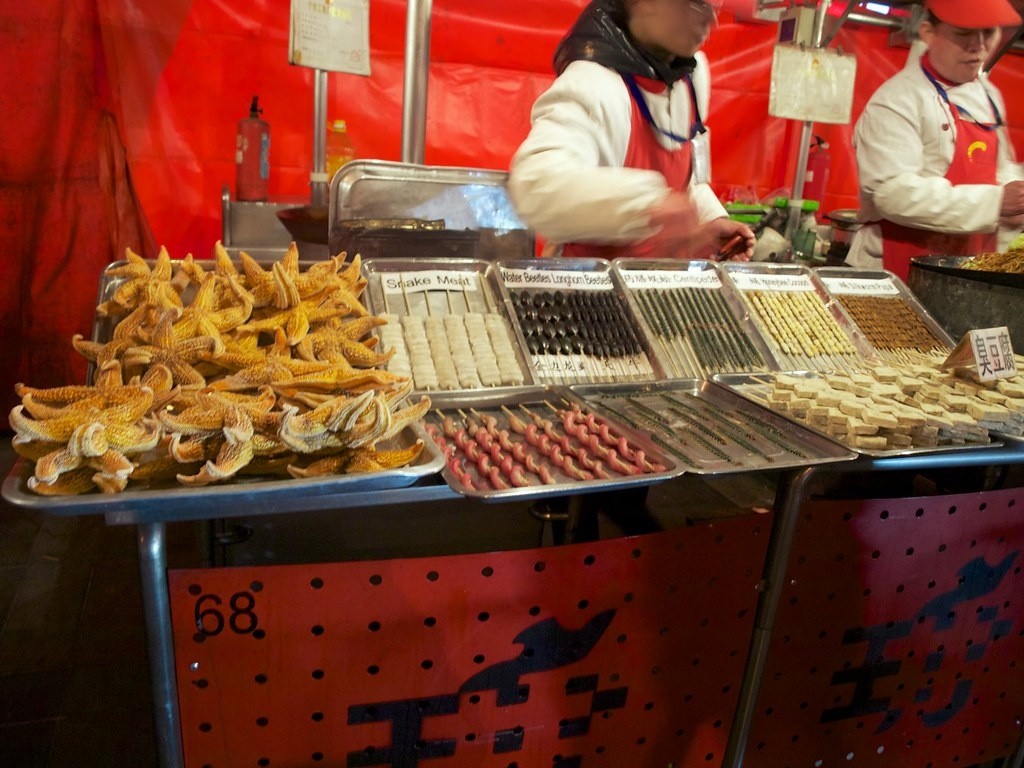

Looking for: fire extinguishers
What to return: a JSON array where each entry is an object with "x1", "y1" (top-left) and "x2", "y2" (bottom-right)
[
  {"x1": 802, "y1": 134, "x2": 834, "y2": 223},
  {"x1": 235, "y1": 93, "x2": 271, "y2": 202}
]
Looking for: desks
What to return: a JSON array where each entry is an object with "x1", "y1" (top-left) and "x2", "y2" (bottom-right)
[{"x1": 104, "y1": 446, "x2": 1024, "y2": 768}]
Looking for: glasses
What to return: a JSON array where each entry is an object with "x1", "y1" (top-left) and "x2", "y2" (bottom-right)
[{"x1": 690, "y1": 0, "x2": 724, "y2": 12}]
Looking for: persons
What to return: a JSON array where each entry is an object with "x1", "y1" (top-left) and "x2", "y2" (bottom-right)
[
  {"x1": 845, "y1": 2, "x2": 1024, "y2": 283},
  {"x1": 506, "y1": 0, "x2": 757, "y2": 264}
]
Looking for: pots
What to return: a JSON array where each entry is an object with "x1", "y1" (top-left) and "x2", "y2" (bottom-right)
[{"x1": 913, "y1": 255, "x2": 1024, "y2": 287}]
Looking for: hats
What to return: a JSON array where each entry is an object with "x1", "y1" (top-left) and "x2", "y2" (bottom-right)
[{"x1": 923, "y1": 0, "x2": 1023, "y2": 28}]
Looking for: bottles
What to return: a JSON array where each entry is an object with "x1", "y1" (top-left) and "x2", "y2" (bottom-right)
[{"x1": 327, "y1": 119, "x2": 353, "y2": 181}]
